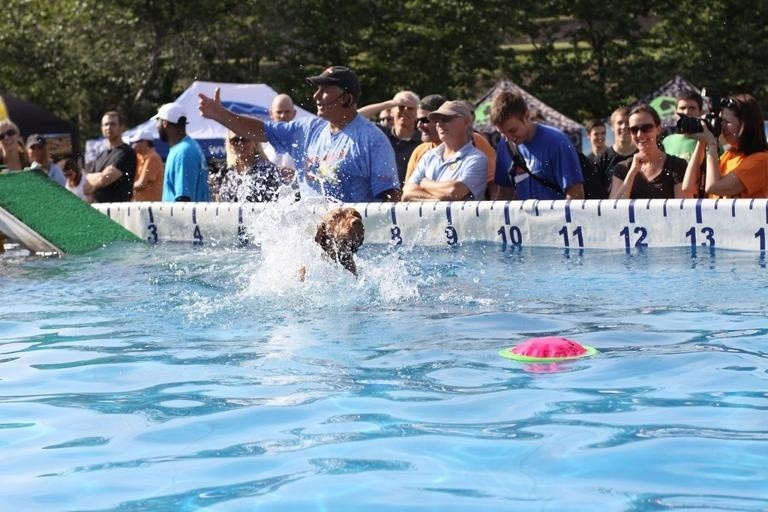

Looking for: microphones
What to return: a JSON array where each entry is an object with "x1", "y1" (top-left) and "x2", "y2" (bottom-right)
[{"x1": 316, "y1": 96, "x2": 343, "y2": 108}]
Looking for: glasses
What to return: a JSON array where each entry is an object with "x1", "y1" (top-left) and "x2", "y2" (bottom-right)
[
  {"x1": 415, "y1": 115, "x2": 431, "y2": 123},
  {"x1": 0, "y1": 129, "x2": 17, "y2": 139},
  {"x1": 227, "y1": 136, "x2": 251, "y2": 144},
  {"x1": 628, "y1": 124, "x2": 655, "y2": 133},
  {"x1": 435, "y1": 117, "x2": 463, "y2": 123}
]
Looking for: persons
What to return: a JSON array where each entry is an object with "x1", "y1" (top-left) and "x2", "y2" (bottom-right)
[{"x1": 0, "y1": 63, "x2": 768, "y2": 199}]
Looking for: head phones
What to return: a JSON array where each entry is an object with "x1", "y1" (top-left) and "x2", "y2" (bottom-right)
[{"x1": 339, "y1": 85, "x2": 355, "y2": 110}]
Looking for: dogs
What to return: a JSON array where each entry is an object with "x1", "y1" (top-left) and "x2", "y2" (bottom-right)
[{"x1": 297, "y1": 207, "x2": 366, "y2": 283}]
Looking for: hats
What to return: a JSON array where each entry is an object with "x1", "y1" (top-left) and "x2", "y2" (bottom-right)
[
  {"x1": 428, "y1": 101, "x2": 471, "y2": 124},
  {"x1": 306, "y1": 66, "x2": 362, "y2": 98},
  {"x1": 129, "y1": 129, "x2": 155, "y2": 142},
  {"x1": 150, "y1": 103, "x2": 190, "y2": 124},
  {"x1": 24, "y1": 134, "x2": 47, "y2": 149}
]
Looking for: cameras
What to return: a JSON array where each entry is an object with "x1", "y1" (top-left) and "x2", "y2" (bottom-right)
[{"x1": 676, "y1": 111, "x2": 723, "y2": 139}]
